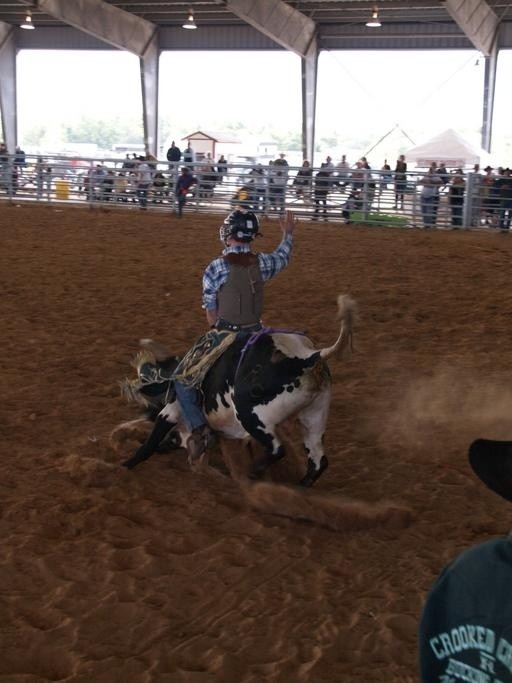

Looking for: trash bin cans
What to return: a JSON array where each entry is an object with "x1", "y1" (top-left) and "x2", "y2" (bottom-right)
[
  {"x1": 55, "y1": 180, "x2": 70, "y2": 200},
  {"x1": 239, "y1": 190, "x2": 248, "y2": 201}
]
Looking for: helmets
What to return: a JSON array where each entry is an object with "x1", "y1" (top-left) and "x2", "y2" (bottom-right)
[{"x1": 219, "y1": 208, "x2": 259, "y2": 247}]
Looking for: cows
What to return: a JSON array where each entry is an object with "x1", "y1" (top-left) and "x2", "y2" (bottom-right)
[{"x1": 120, "y1": 291, "x2": 359, "y2": 489}]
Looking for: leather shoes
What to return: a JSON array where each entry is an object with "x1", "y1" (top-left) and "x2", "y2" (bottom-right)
[{"x1": 186, "y1": 424, "x2": 218, "y2": 462}]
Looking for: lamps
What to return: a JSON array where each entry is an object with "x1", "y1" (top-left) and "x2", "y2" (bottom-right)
[
  {"x1": 182, "y1": 0, "x2": 198, "y2": 29},
  {"x1": 365, "y1": 0, "x2": 382, "y2": 27},
  {"x1": 20, "y1": 7, "x2": 35, "y2": 29}
]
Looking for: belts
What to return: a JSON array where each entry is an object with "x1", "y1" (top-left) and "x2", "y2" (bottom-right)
[{"x1": 224, "y1": 322, "x2": 257, "y2": 329}]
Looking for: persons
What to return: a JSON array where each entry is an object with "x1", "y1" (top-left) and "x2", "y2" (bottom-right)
[
  {"x1": 238, "y1": 153, "x2": 375, "y2": 224},
  {"x1": 1, "y1": 138, "x2": 511, "y2": 234},
  {"x1": 415, "y1": 161, "x2": 510, "y2": 229},
  {"x1": 379, "y1": 155, "x2": 407, "y2": 209},
  {"x1": 168, "y1": 207, "x2": 297, "y2": 467},
  {"x1": 155, "y1": 141, "x2": 228, "y2": 216},
  {"x1": 1, "y1": 144, "x2": 26, "y2": 192},
  {"x1": 412, "y1": 436, "x2": 511, "y2": 682},
  {"x1": 83, "y1": 152, "x2": 152, "y2": 211}
]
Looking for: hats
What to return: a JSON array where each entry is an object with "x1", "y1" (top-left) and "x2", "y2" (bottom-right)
[{"x1": 469, "y1": 438, "x2": 512, "y2": 502}]
